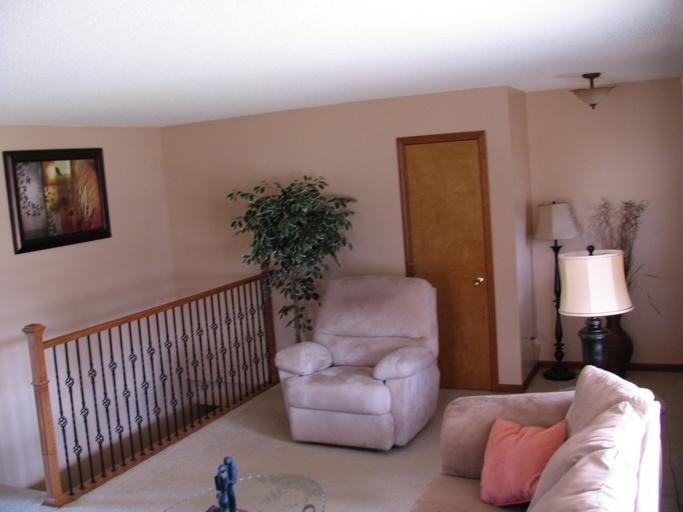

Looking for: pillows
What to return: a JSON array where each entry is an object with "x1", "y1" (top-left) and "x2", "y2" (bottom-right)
[{"x1": 479, "y1": 418, "x2": 571, "y2": 506}]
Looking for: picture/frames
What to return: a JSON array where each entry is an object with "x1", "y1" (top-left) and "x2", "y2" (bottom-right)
[{"x1": 3, "y1": 145, "x2": 114, "y2": 256}]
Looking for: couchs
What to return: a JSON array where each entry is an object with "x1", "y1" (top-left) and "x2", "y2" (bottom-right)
[{"x1": 408, "y1": 364, "x2": 669, "y2": 512}]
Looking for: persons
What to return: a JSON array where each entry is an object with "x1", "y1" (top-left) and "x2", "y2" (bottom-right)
[{"x1": 215, "y1": 457, "x2": 238, "y2": 512}]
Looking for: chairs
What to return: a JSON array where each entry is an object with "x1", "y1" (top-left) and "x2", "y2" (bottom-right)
[{"x1": 273, "y1": 275, "x2": 444, "y2": 455}]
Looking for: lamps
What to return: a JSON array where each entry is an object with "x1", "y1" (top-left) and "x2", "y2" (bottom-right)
[{"x1": 532, "y1": 200, "x2": 634, "y2": 382}]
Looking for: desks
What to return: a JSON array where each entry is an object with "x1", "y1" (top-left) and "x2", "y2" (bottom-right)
[{"x1": 162, "y1": 469, "x2": 329, "y2": 512}]
